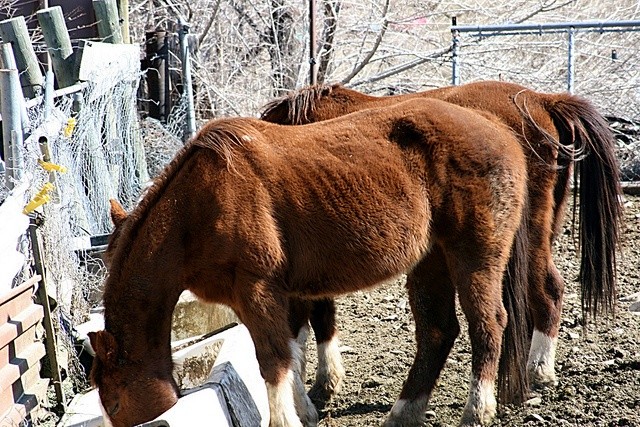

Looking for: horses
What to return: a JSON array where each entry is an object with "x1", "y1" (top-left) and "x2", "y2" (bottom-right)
[
  {"x1": 87, "y1": 95, "x2": 546, "y2": 427},
  {"x1": 103, "y1": 80, "x2": 625, "y2": 408}
]
